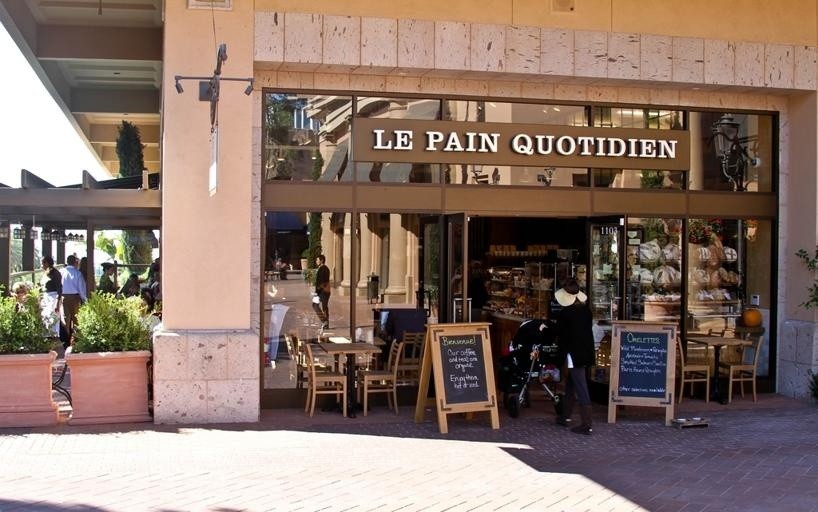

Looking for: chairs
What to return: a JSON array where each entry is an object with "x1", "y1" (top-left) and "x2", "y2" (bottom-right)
[
  {"x1": 676, "y1": 334, "x2": 711, "y2": 405},
  {"x1": 688, "y1": 328, "x2": 725, "y2": 396},
  {"x1": 51, "y1": 336, "x2": 73, "y2": 408},
  {"x1": 284, "y1": 330, "x2": 426, "y2": 418},
  {"x1": 717, "y1": 331, "x2": 764, "y2": 403}
]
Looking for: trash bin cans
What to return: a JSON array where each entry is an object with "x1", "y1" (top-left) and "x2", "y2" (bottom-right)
[{"x1": 367, "y1": 272, "x2": 379, "y2": 304}]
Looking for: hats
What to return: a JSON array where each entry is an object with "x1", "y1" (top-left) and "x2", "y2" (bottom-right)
[{"x1": 555, "y1": 281, "x2": 589, "y2": 308}]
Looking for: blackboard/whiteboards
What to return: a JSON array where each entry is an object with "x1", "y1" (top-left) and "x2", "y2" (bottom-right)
[
  {"x1": 430, "y1": 323, "x2": 497, "y2": 413},
  {"x1": 609, "y1": 321, "x2": 679, "y2": 407}
]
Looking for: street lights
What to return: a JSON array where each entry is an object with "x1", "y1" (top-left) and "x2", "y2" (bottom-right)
[{"x1": 701, "y1": 110, "x2": 759, "y2": 350}]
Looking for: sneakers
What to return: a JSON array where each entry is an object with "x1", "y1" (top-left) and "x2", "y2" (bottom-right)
[{"x1": 320, "y1": 321, "x2": 331, "y2": 331}]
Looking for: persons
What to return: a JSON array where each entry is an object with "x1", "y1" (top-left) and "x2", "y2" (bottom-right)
[
  {"x1": 312, "y1": 254, "x2": 330, "y2": 329},
  {"x1": 276, "y1": 258, "x2": 288, "y2": 280},
  {"x1": 12, "y1": 282, "x2": 28, "y2": 311},
  {"x1": 38, "y1": 254, "x2": 87, "y2": 343},
  {"x1": 540, "y1": 278, "x2": 594, "y2": 435},
  {"x1": 129, "y1": 258, "x2": 161, "y2": 313},
  {"x1": 98, "y1": 263, "x2": 117, "y2": 293}
]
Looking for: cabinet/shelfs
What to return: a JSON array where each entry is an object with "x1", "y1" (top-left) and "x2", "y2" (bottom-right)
[
  {"x1": 643, "y1": 299, "x2": 740, "y2": 336},
  {"x1": 523, "y1": 260, "x2": 558, "y2": 320},
  {"x1": 489, "y1": 266, "x2": 523, "y2": 315}
]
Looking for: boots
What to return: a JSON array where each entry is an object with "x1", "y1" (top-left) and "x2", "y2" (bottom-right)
[
  {"x1": 572, "y1": 405, "x2": 595, "y2": 436},
  {"x1": 556, "y1": 398, "x2": 576, "y2": 428}
]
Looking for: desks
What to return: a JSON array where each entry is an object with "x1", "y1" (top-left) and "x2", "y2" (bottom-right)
[{"x1": 686, "y1": 333, "x2": 754, "y2": 405}]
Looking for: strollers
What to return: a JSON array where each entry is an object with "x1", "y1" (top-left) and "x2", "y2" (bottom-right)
[{"x1": 491, "y1": 318, "x2": 564, "y2": 418}]
